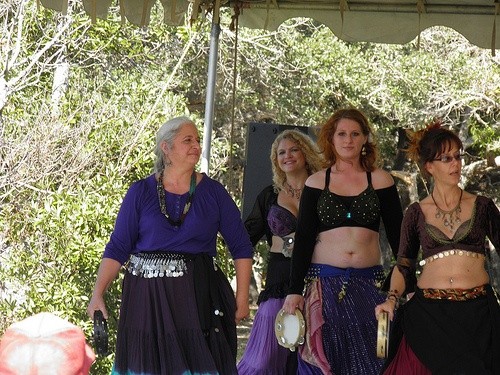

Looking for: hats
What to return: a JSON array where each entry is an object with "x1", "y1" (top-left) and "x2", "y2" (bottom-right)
[{"x1": 0, "y1": 312, "x2": 96, "y2": 375}]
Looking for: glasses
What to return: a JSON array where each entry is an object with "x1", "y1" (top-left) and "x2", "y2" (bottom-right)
[{"x1": 431, "y1": 153, "x2": 463, "y2": 163}]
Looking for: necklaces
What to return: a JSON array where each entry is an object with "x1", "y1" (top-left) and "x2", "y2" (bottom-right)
[
  {"x1": 286, "y1": 182, "x2": 303, "y2": 199},
  {"x1": 432, "y1": 188, "x2": 462, "y2": 226}
]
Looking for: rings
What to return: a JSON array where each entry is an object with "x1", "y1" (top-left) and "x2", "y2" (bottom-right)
[{"x1": 244, "y1": 318, "x2": 246, "y2": 320}]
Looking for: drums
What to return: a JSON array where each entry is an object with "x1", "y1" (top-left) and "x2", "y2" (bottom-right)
[
  {"x1": 273, "y1": 305, "x2": 306, "y2": 353},
  {"x1": 375, "y1": 311, "x2": 390, "y2": 360},
  {"x1": 93, "y1": 310, "x2": 110, "y2": 355}
]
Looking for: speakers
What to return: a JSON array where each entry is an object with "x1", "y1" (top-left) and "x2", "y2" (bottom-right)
[{"x1": 239, "y1": 122, "x2": 309, "y2": 222}]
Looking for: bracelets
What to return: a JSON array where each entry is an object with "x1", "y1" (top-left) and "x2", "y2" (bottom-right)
[{"x1": 386, "y1": 289, "x2": 400, "y2": 310}]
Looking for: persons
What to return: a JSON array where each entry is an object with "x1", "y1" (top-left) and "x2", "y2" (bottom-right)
[
  {"x1": 282, "y1": 106, "x2": 417, "y2": 375},
  {"x1": 373, "y1": 120, "x2": 500, "y2": 375},
  {"x1": 85, "y1": 116, "x2": 254, "y2": 375},
  {"x1": 237, "y1": 129, "x2": 331, "y2": 375},
  {"x1": 0, "y1": 311, "x2": 96, "y2": 375}
]
